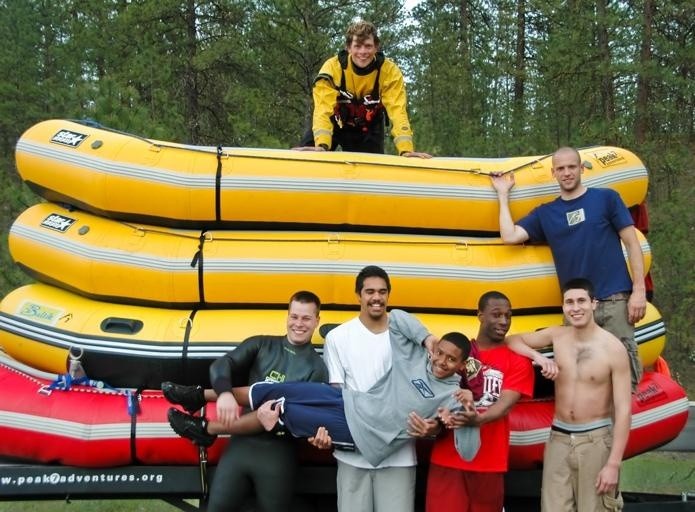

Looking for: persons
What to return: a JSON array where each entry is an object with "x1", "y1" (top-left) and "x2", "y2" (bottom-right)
[
  {"x1": 290, "y1": 22, "x2": 431, "y2": 159},
  {"x1": 311, "y1": 265, "x2": 441, "y2": 511},
  {"x1": 489, "y1": 147, "x2": 646, "y2": 395},
  {"x1": 505, "y1": 279, "x2": 634, "y2": 511},
  {"x1": 161, "y1": 309, "x2": 474, "y2": 466},
  {"x1": 425, "y1": 291, "x2": 536, "y2": 511},
  {"x1": 207, "y1": 293, "x2": 328, "y2": 510}
]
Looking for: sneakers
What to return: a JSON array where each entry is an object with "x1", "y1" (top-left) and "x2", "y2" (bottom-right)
[{"x1": 161, "y1": 382, "x2": 217, "y2": 447}]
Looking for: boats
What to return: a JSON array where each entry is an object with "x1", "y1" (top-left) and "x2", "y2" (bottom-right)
[
  {"x1": 6, "y1": 200, "x2": 654, "y2": 308},
  {"x1": 1, "y1": 350, "x2": 690, "y2": 469},
  {"x1": 1, "y1": 283, "x2": 666, "y2": 387},
  {"x1": 13, "y1": 116, "x2": 651, "y2": 236}
]
